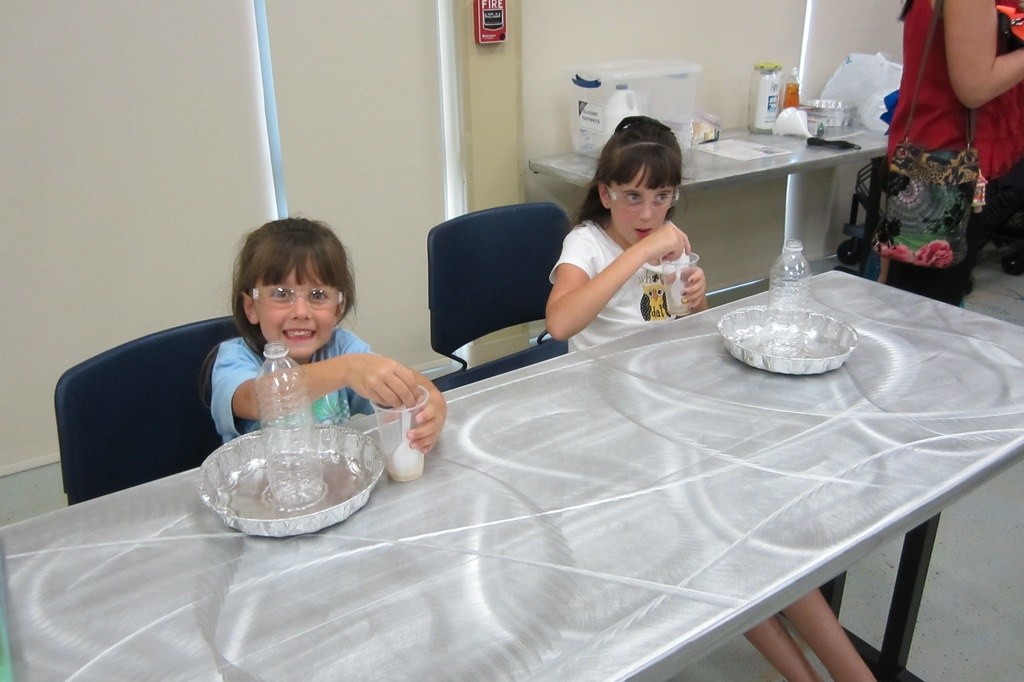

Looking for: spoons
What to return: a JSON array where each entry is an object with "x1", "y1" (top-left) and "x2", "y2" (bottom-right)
[
  {"x1": 393, "y1": 405, "x2": 419, "y2": 473},
  {"x1": 671, "y1": 257, "x2": 685, "y2": 306}
]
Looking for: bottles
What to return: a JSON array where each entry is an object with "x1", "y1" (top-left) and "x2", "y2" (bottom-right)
[
  {"x1": 607, "y1": 85, "x2": 640, "y2": 141},
  {"x1": 764, "y1": 239, "x2": 811, "y2": 357},
  {"x1": 783, "y1": 67, "x2": 800, "y2": 109},
  {"x1": 255, "y1": 341, "x2": 323, "y2": 509}
]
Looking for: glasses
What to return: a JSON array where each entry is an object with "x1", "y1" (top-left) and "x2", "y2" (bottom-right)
[
  {"x1": 603, "y1": 183, "x2": 680, "y2": 212},
  {"x1": 258, "y1": 284, "x2": 347, "y2": 310}
]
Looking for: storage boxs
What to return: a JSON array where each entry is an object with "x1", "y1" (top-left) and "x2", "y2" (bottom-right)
[{"x1": 572, "y1": 56, "x2": 704, "y2": 158}]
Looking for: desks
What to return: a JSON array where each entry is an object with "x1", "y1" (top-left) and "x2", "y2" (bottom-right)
[
  {"x1": 0, "y1": 269, "x2": 1023, "y2": 682},
  {"x1": 527, "y1": 125, "x2": 891, "y2": 195}
]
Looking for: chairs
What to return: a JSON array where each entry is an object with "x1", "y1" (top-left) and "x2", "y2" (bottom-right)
[
  {"x1": 54, "y1": 316, "x2": 242, "y2": 505},
  {"x1": 427, "y1": 200, "x2": 573, "y2": 385}
]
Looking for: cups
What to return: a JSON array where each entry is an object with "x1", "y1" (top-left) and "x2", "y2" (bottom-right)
[
  {"x1": 748, "y1": 62, "x2": 784, "y2": 135},
  {"x1": 660, "y1": 252, "x2": 699, "y2": 316},
  {"x1": 370, "y1": 385, "x2": 429, "y2": 481}
]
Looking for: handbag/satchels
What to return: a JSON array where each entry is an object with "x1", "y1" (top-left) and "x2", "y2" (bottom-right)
[{"x1": 871, "y1": 138, "x2": 979, "y2": 271}]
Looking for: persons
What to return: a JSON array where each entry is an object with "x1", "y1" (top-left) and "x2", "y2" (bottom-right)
[
  {"x1": 887, "y1": 0, "x2": 1024, "y2": 304},
  {"x1": 209, "y1": 217, "x2": 447, "y2": 453},
  {"x1": 546, "y1": 115, "x2": 877, "y2": 682}
]
